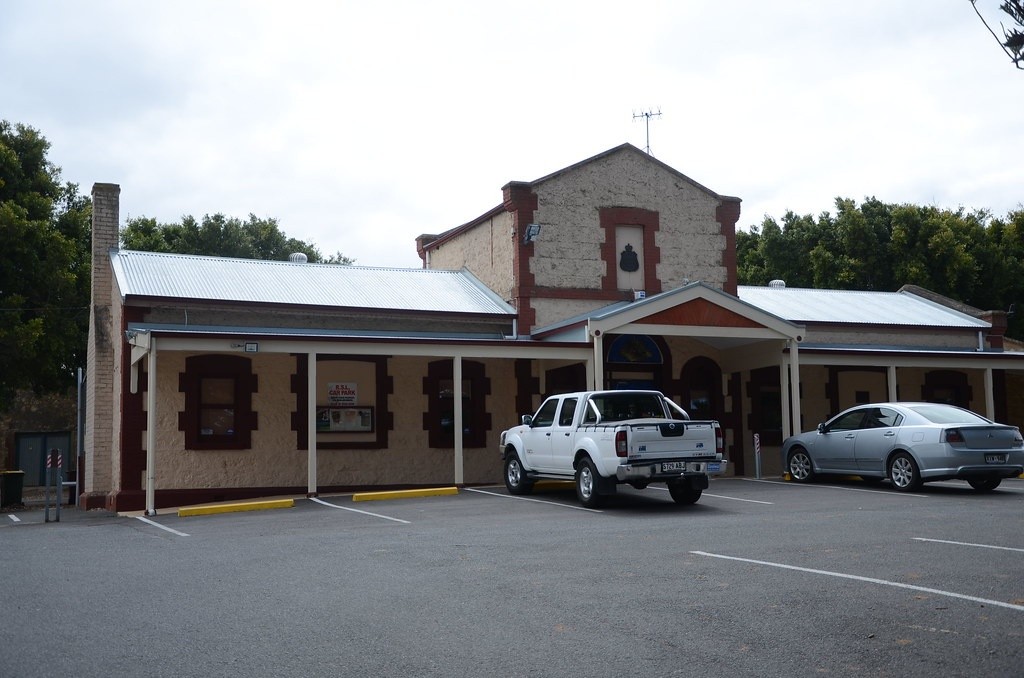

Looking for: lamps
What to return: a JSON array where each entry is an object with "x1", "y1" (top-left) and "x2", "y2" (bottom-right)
[{"x1": 523, "y1": 224, "x2": 541, "y2": 241}]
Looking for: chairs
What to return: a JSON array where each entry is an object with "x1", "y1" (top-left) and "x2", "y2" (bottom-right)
[
  {"x1": 559, "y1": 400, "x2": 590, "y2": 425},
  {"x1": 603, "y1": 396, "x2": 632, "y2": 420}
]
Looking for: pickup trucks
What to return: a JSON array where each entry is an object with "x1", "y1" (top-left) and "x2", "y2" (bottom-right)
[{"x1": 498, "y1": 390, "x2": 727, "y2": 513}]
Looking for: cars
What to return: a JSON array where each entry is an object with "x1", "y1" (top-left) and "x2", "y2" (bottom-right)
[{"x1": 779, "y1": 397, "x2": 1024, "y2": 493}]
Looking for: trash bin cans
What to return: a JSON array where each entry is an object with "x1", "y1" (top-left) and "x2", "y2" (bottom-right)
[{"x1": 1, "y1": 468, "x2": 27, "y2": 511}]
